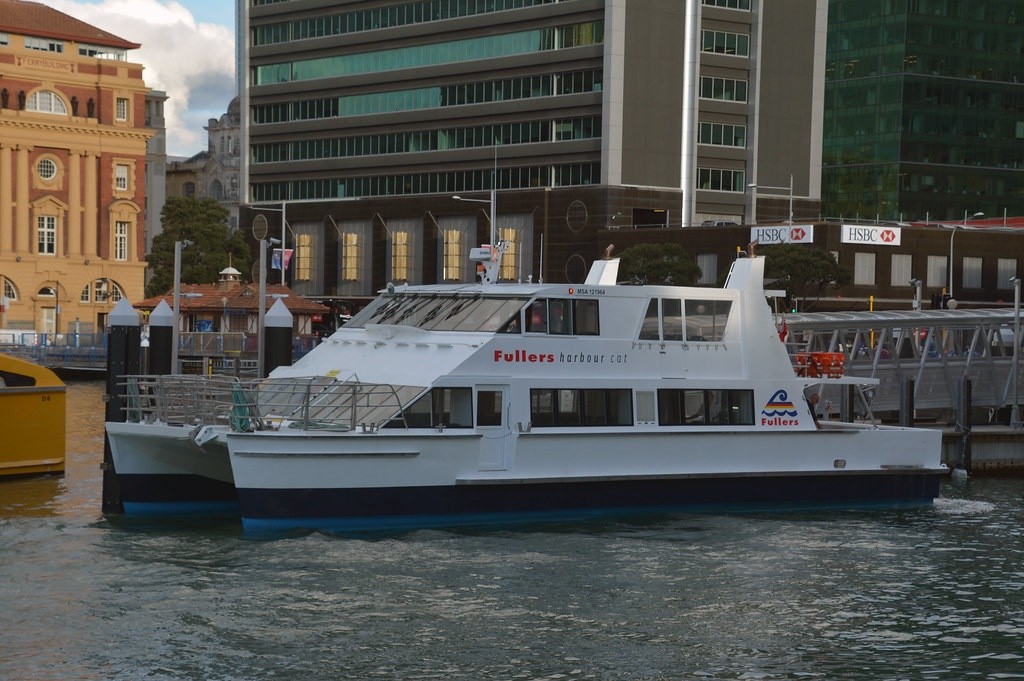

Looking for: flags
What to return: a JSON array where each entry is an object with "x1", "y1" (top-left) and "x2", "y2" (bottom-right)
[{"x1": 778, "y1": 319, "x2": 789, "y2": 344}]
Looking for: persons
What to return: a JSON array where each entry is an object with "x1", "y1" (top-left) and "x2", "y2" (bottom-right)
[
  {"x1": 806, "y1": 394, "x2": 823, "y2": 430},
  {"x1": 685, "y1": 392, "x2": 719, "y2": 424}
]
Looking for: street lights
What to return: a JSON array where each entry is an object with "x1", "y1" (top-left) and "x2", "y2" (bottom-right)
[
  {"x1": 452, "y1": 195, "x2": 496, "y2": 253},
  {"x1": 947, "y1": 213, "x2": 984, "y2": 309},
  {"x1": 246, "y1": 205, "x2": 286, "y2": 286},
  {"x1": 749, "y1": 184, "x2": 793, "y2": 244}
]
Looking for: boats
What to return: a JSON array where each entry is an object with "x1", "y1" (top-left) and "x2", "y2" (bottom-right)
[
  {"x1": 1, "y1": 352, "x2": 68, "y2": 481},
  {"x1": 104, "y1": 244, "x2": 951, "y2": 543}
]
